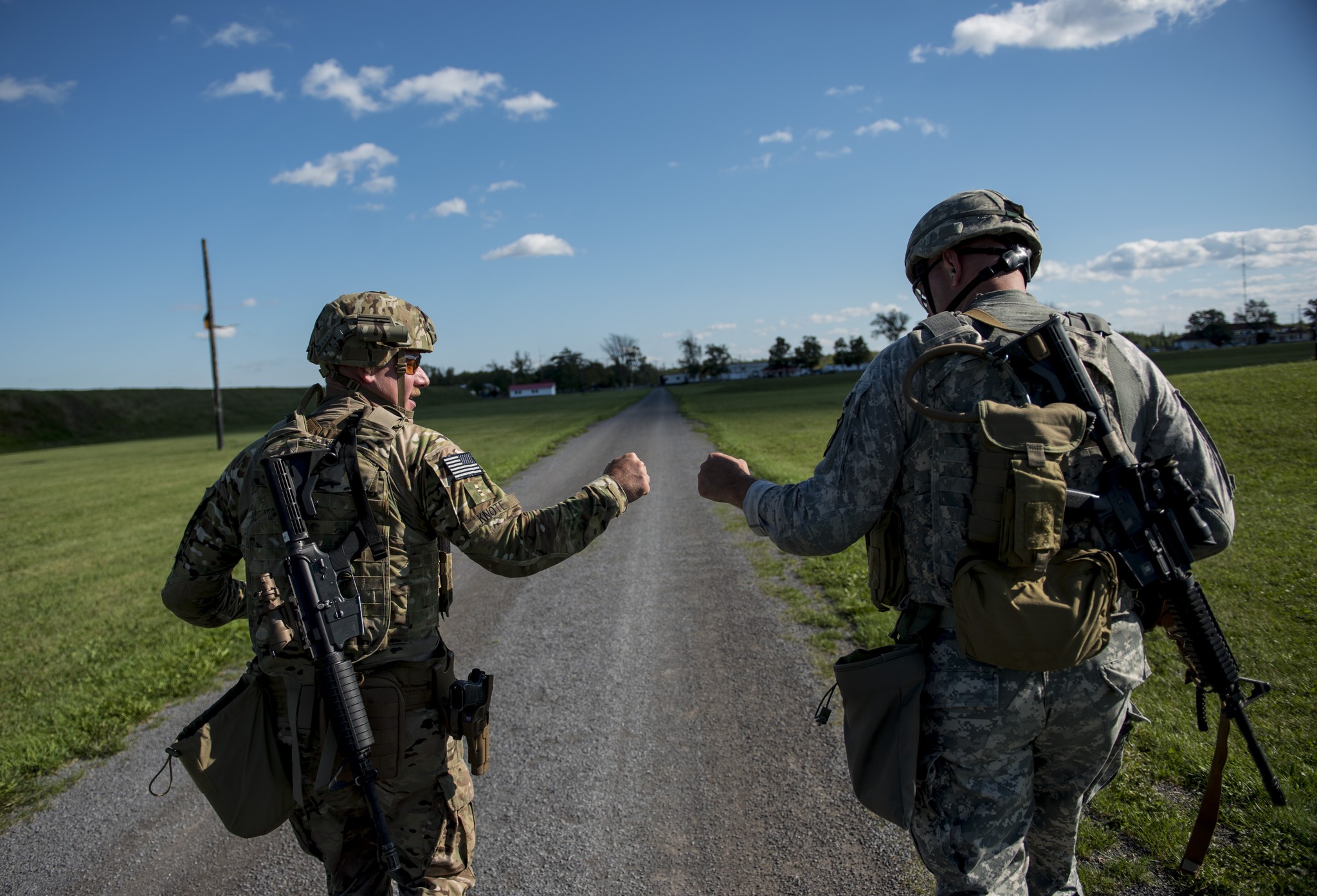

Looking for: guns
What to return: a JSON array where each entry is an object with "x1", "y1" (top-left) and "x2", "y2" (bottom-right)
[
  {"x1": 999, "y1": 309, "x2": 1287, "y2": 811},
  {"x1": 260, "y1": 457, "x2": 416, "y2": 875}
]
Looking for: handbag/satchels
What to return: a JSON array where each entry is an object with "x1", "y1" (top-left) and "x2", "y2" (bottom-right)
[
  {"x1": 812, "y1": 640, "x2": 931, "y2": 830},
  {"x1": 148, "y1": 665, "x2": 298, "y2": 839}
]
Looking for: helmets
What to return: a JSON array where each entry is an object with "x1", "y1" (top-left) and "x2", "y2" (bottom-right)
[
  {"x1": 305, "y1": 291, "x2": 437, "y2": 370},
  {"x1": 905, "y1": 189, "x2": 1042, "y2": 284}
]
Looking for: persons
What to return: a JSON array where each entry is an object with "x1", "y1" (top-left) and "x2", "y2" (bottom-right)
[
  {"x1": 695, "y1": 184, "x2": 1237, "y2": 896},
  {"x1": 161, "y1": 285, "x2": 653, "y2": 896}
]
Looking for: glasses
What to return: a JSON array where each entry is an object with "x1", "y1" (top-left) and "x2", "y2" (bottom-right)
[
  {"x1": 402, "y1": 351, "x2": 422, "y2": 374},
  {"x1": 913, "y1": 258, "x2": 939, "y2": 312}
]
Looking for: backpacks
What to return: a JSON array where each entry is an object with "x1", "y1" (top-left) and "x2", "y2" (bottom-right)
[{"x1": 951, "y1": 401, "x2": 1120, "y2": 673}]
